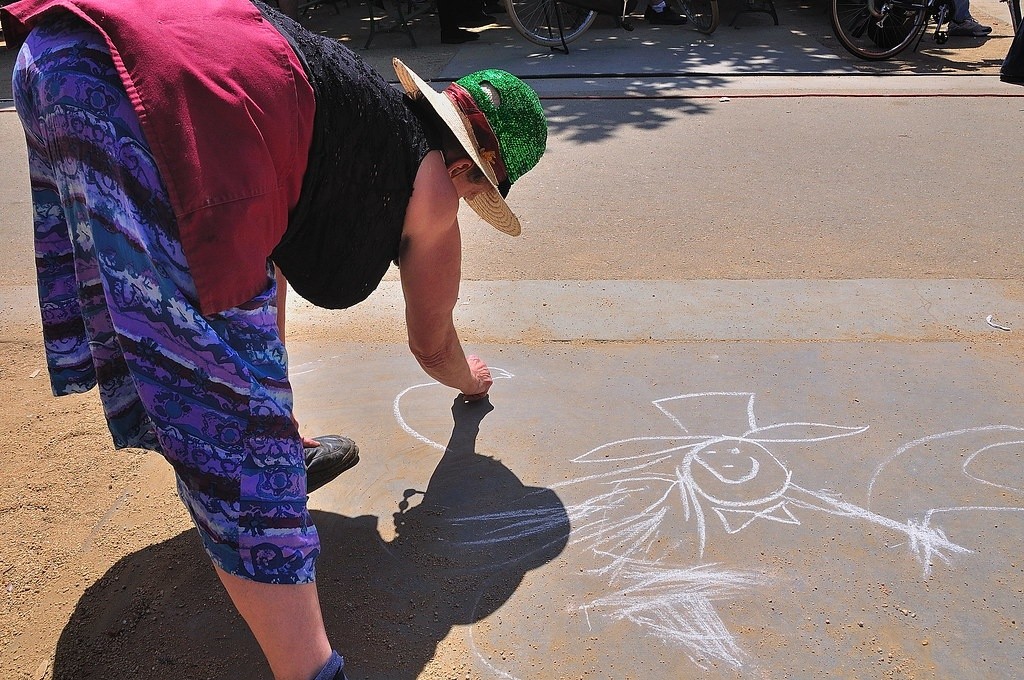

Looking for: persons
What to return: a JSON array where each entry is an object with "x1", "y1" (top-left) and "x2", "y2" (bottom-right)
[
  {"x1": 645, "y1": 0, "x2": 687, "y2": 25},
  {"x1": 947, "y1": 0, "x2": 992, "y2": 36},
  {"x1": 436, "y1": 0, "x2": 506, "y2": 44},
  {"x1": 0, "y1": 0, "x2": 549, "y2": 680}
]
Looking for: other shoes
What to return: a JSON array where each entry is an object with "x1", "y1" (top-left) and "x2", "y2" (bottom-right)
[{"x1": 644, "y1": 4, "x2": 687, "y2": 24}]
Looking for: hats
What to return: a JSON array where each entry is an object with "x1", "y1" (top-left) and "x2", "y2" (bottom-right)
[{"x1": 393, "y1": 56, "x2": 549, "y2": 235}]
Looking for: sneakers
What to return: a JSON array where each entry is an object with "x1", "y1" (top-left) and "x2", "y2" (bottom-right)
[{"x1": 948, "y1": 16, "x2": 992, "y2": 36}]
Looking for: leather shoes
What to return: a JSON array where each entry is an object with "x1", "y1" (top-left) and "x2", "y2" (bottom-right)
[{"x1": 301, "y1": 434, "x2": 358, "y2": 487}]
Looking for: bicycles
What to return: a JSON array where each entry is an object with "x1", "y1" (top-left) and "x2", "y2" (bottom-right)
[
  {"x1": 830, "y1": 0, "x2": 957, "y2": 62},
  {"x1": 503, "y1": 0, "x2": 721, "y2": 56}
]
